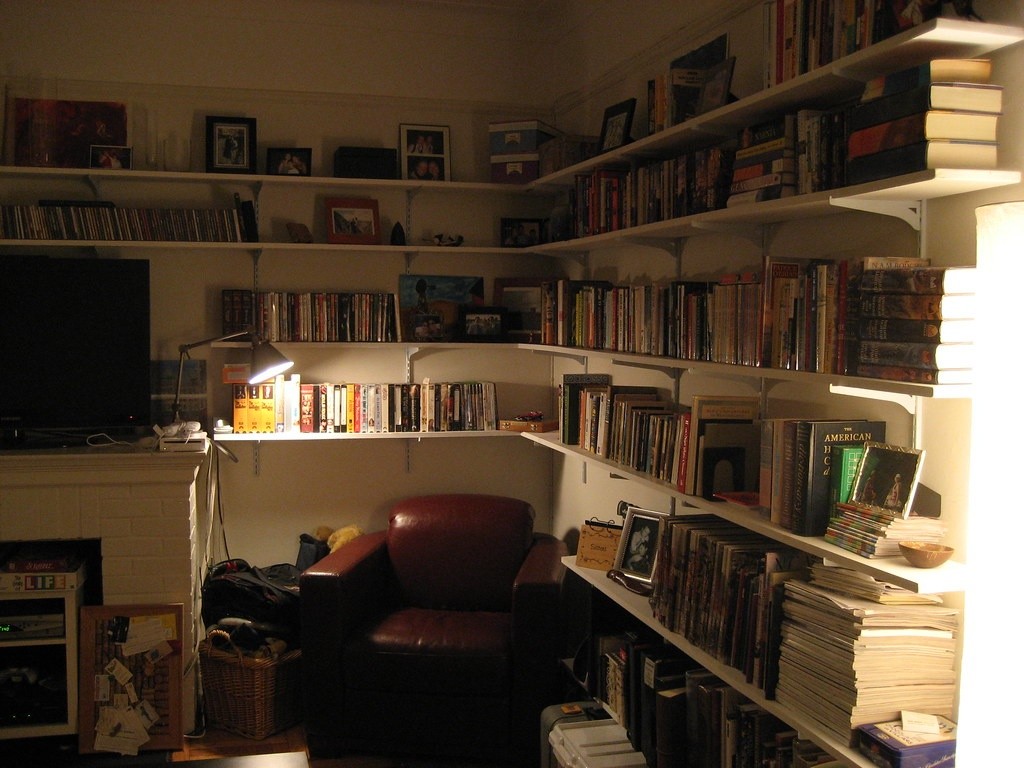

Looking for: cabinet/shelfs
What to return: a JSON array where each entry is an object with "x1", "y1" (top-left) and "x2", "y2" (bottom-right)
[{"x1": 0, "y1": 21, "x2": 1024, "y2": 768}]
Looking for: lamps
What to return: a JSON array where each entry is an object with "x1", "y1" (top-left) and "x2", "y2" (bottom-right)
[{"x1": 158, "y1": 324, "x2": 295, "y2": 452}]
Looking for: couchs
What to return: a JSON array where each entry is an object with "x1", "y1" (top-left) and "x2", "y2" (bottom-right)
[{"x1": 297, "y1": 493, "x2": 569, "y2": 768}]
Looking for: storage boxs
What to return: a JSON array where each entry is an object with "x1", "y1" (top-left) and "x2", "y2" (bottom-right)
[
  {"x1": 496, "y1": 417, "x2": 559, "y2": 433},
  {"x1": 548, "y1": 719, "x2": 648, "y2": 768},
  {"x1": 856, "y1": 714, "x2": 957, "y2": 768},
  {"x1": 0, "y1": 558, "x2": 89, "y2": 591},
  {"x1": 333, "y1": 146, "x2": 397, "y2": 180},
  {"x1": 487, "y1": 118, "x2": 600, "y2": 185}
]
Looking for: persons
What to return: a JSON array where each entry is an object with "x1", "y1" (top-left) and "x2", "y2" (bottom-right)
[
  {"x1": 624, "y1": 521, "x2": 653, "y2": 572},
  {"x1": 407, "y1": 134, "x2": 444, "y2": 181},
  {"x1": 466, "y1": 316, "x2": 500, "y2": 336},
  {"x1": 858, "y1": 470, "x2": 878, "y2": 505},
  {"x1": 883, "y1": 474, "x2": 903, "y2": 510},
  {"x1": 222, "y1": 135, "x2": 238, "y2": 164},
  {"x1": 505, "y1": 223, "x2": 539, "y2": 243},
  {"x1": 350, "y1": 217, "x2": 362, "y2": 234},
  {"x1": 279, "y1": 154, "x2": 306, "y2": 174},
  {"x1": 416, "y1": 320, "x2": 439, "y2": 338}
]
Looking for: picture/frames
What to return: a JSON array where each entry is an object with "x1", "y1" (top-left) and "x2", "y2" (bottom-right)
[
  {"x1": 492, "y1": 276, "x2": 569, "y2": 344},
  {"x1": 324, "y1": 196, "x2": 384, "y2": 246},
  {"x1": 598, "y1": 100, "x2": 637, "y2": 155},
  {"x1": 409, "y1": 313, "x2": 444, "y2": 342},
  {"x1": 265, "y1": 147, "x2": 313, "y2": 177},
  {"x1": 847, "y1": 439, "x2": 926, "y2": 521},
  {"x1": 695, "y1": 56, "x2": 736, "y2": 118},
  {"x1": 89, "y1": 145, "x2": 133, "y2": 171},
  {"x1": 612, "y1": 506, "x2": 672, "y2": 586},
  {"x1": 205, "y1": 115, "x2": 257, "y2": 175},
  {"x1": 457, "y1": 304, "x2": 508, "y2": 343},
  {"x1": 399, "y1": 123, "x2": 452, "y2": 184},
  {"x1": 501, "y1": 217, "x2": 543, "y2": 248}
]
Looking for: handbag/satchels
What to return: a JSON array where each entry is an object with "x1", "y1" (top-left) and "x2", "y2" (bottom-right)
[
  {"x1": 201, "y1": 557, "x2": 307, "y2": 651},
  {"x1": 296, "y1": 534, "x2": 330, "y2": 572}
]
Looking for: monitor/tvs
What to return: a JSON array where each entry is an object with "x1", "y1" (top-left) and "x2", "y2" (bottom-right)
[{"x1": 0, "y1": 254, "x2": 155, "y2": 450}]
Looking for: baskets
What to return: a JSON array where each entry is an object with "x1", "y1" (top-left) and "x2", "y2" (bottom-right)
[{"x1": 197, "y1": 629, "x2": 306, "y2": 740}]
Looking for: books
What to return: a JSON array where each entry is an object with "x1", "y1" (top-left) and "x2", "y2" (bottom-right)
[
  {"x1": 222, "y1": 288, "x2": 398, "y2": 342},
  {"x1": 558, "y1": 374, "x2": 949, "y2": 559},
  {"x1": 541, "y1": 59, "x2": 1004, "y2": 387},
  {"x1": 596, "y1": 628, "x2": 842, "y2": 768},
  {"x1": 0, "y1": 200, "x2": 258, "y2": 243},
  {"x1": 650, "y1": 516, "x2": 810, "y2": 702},
  {"x1": 762, "y1": 0, "x2": 923, "y2": 90},
  {"x1": 299, "y1": 380, "x2": 499, "y2": 432}
]
